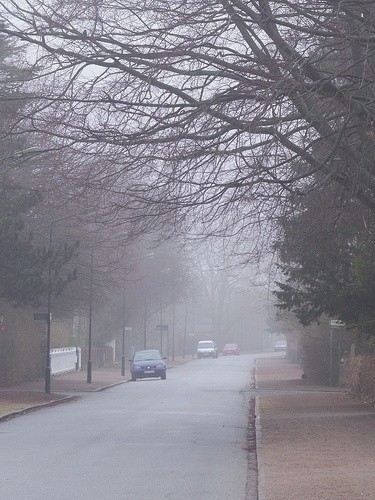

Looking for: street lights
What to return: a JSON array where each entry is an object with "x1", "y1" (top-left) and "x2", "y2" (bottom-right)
[
  {"x1": 44, "y1": 207, "x2": 95, "y2": 393},
  {"x1": 87, "y1": 231, "x2": 194, "y2": 383}
]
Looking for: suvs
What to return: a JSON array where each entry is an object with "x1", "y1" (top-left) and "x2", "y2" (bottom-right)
[{"x1": 197, "y1": 341, "x2": 218, "y2": 359}]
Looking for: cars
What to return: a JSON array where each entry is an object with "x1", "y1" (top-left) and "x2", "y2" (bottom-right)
[
  {"x1": 223, "y1": 343, "x2": 240, "y2": 356},
  {"x1": 127, "y1": 350, "x2": 168, "y2": 380},
  {"x1": 275, "y1": 341, "x2": 287, "y2": 352}
]
